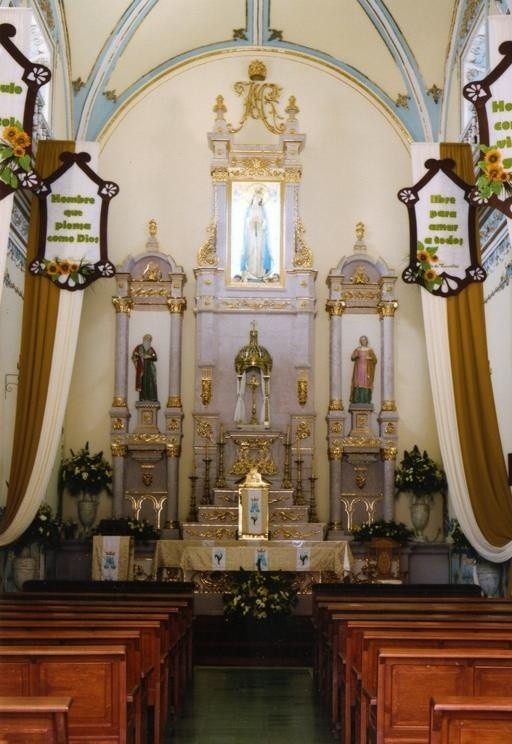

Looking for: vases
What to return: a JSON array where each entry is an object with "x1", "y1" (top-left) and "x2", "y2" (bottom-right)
[
  {"x1": 411, "y1": 496, "x2": 431, "y2": 542},
  {"x1": 78, "y1": 491, "x2": 98, "y2": 534}
]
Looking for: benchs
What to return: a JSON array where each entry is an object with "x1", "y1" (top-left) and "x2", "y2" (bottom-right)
[
  {"x1": 0, "y1": 580, "x2": 195, "y2": 744},
  {"x1": 309, "y1": 581, "x2": 512, "y2": 744}
]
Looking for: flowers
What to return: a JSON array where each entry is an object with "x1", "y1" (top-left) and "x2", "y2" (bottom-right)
[
  {"x1": 63, "y1": 441, "x2": 112, "y2": 494},
  {"x1": 394, "y1": 445, "x2": 444, "y2": 497}
]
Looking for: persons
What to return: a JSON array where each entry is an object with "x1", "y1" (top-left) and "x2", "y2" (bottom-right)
[
  {"x1": 239, "y1": 187, "x2": 273, "y2": 276},
  {"x1": 132, "y1": 333, "x2": 158, "y2": 402},
  {"x1": 350, "y1": 335, "x2": 378, "y2": 402}
]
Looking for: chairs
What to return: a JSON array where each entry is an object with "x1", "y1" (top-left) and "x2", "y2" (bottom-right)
[{"x1": 366, "y1": 538, "x2": 403, "y2": 586}]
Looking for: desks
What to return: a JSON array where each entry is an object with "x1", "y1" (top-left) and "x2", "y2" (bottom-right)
[{"x1": 154, "y1": 540, "x2": 355, "y2": 616}]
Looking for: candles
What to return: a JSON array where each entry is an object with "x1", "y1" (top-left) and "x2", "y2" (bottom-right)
[{"x1": 192, "y1": 424, "x2": 315, "y2": 478}]
